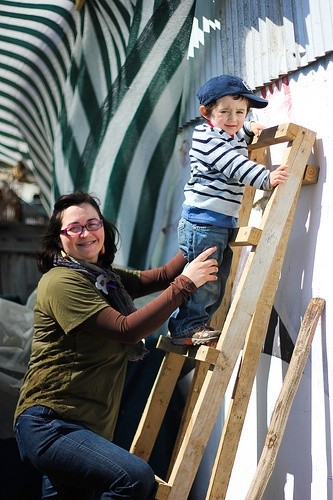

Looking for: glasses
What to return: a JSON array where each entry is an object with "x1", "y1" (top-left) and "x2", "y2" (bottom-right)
[{"x1": 59, "y1": 219, "x2": 103, "y2": 237}]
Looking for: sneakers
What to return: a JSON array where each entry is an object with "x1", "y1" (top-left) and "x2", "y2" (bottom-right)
[{"x1": 171, "y1": 325, "x2": 221, "y2": 346}]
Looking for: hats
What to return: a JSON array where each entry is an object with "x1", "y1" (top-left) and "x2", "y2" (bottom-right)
[{"x1": 196, "y1": 75, "x2": 269, "y2": 108}]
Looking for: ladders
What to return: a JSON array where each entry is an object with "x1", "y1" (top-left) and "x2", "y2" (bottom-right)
[{"x1": 128, "y1": 123, "x2": 316, "y2": 500}]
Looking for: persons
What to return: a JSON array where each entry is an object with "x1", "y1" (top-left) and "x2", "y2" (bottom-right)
[
  {"x1": 14, "y1": 191, "x2": 218, "y2": 500},
  {"x1": 167, "y1": 75, "x2": 289, "y2": 345}
]
